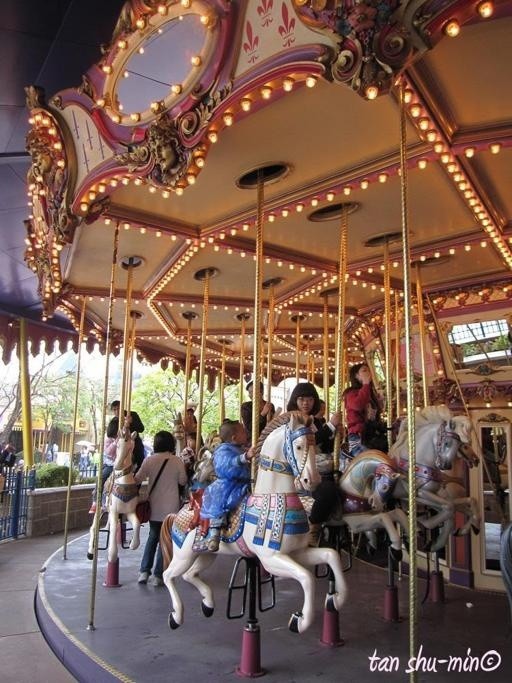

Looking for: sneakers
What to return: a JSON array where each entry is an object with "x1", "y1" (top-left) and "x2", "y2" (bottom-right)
[
  {"x1": 88, "y1": 505, "x2": 96, "y2": 514},
  {"x1": 308, "y1": 524, "x2": 321, "y2": 547},
  {"x1": 209, "y1": 536, "x2": 219, "y2": 552},
  {"x1": 138, "y1": 572, "x2": 149, "y2": 584},
  {"x1": 153, "y1": 578, "x2": 163, "y2": 586}
]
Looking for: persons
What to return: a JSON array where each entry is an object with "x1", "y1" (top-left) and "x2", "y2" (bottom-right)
[
  {"x1": 182, "y1": 363, "x2": 388, "y2": 553},
  {"x1": 0, "y1": 399, "x2": 147, "y2": 515},
  {"x1": 134, "y1": 429, "x2": 188, "y2": 588}
]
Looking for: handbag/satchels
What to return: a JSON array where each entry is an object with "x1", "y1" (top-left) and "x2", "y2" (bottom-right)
[
  {"x1": 136, "y1": 500, "x2": 151, "y2": 523},
  {"x1": 362, "y1": 420, "x2": 387, "y2": 452}
]
